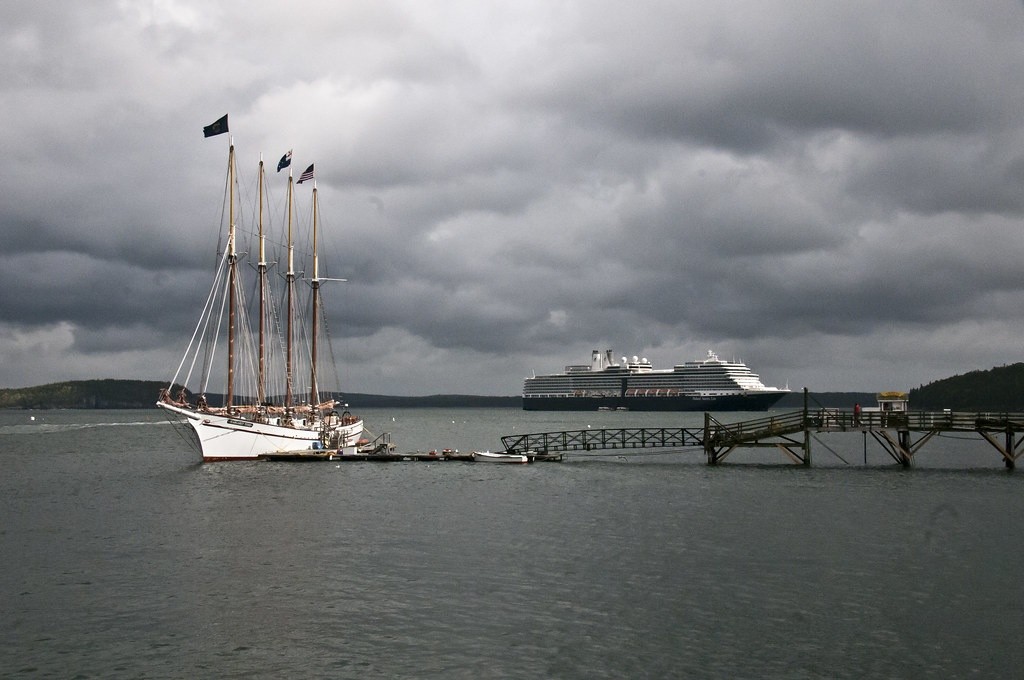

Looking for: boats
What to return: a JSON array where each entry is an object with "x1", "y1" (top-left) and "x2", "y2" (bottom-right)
[
  {"x1": 852, "y1": 398, "x2": 909, "y2": 425},
  {"x1": 473, "y1": 449, "x2": 539, "y2": 465},
  {"x1": 808, "y1": 407, "x2": 840, "y2": 426}
]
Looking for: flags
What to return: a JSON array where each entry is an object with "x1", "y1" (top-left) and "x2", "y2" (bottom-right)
[
  {"x1": 278, "y1": 149, "x2": 292, "y2": 172},
  {"x1": 204, "y1": 114, "x2": 229, "y2": 138},
  {"x1": 297, "y1": 164, "x2": 314, "y2": 184}
]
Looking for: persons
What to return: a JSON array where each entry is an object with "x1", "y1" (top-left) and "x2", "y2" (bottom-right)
[
  {"x1": 855, "y1": 403, "x2": 861, "y2": 427},
  {"x1": 202, "y1": 394, "x2": 206, "y2": 406}
]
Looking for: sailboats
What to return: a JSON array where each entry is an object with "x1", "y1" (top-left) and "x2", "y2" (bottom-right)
[{"x1": 155, "y1": 136, "x2": 366, "y2": 463}]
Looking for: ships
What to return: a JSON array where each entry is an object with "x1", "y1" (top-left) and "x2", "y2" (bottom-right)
[{"x1": 521, "y1": 349, "x2": 792, "y2": 411}]
